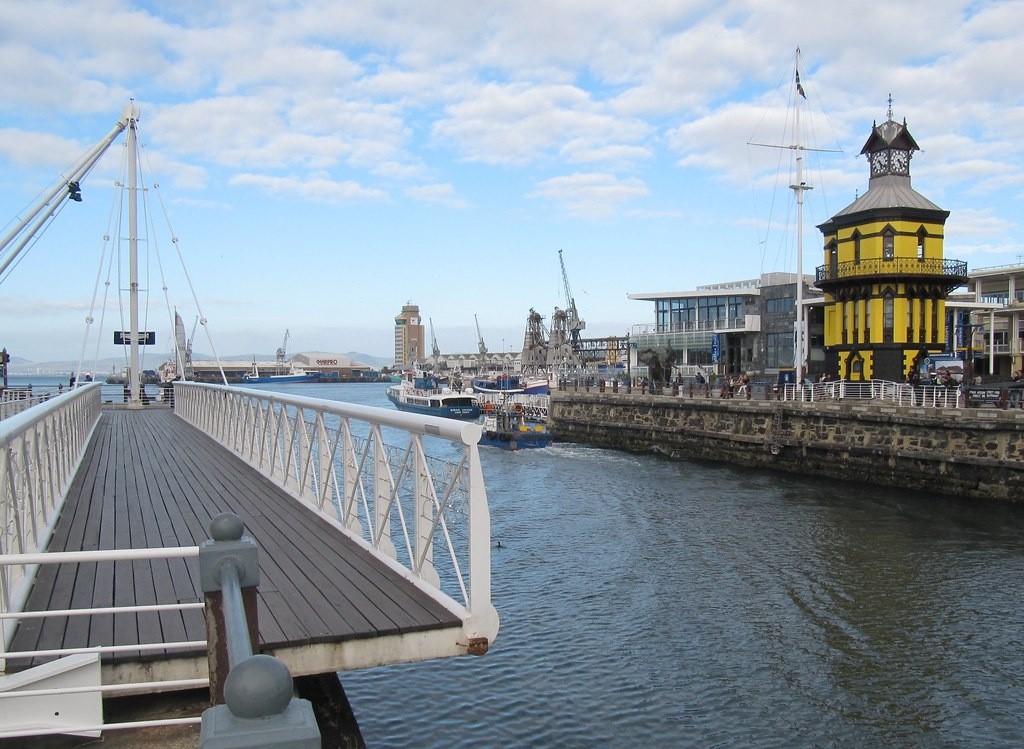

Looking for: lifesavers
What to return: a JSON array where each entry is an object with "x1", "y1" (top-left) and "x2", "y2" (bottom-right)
[{"x1": 514, "y1": 404, "x2": 522, "y2": 413}]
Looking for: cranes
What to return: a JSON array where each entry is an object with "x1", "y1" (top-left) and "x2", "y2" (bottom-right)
[
  {"x1": 475, "y1": 313, "x2": 488, "y2": 362},
  {"x1": 429, "y1": 317, "x2": 441, "y2": 363},
  {"x1": 276, "y1": 328, "x2": 292, "y2": 376},
  {"x1": 558, "y1": 248, "x2": 586, "y2": 351}
]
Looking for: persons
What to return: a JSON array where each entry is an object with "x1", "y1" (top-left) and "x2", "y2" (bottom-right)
[
  {"x1": 68, "y1": 374, "x2": 78, "y2": 389},
  {"x1": 720, "y1": 370, "x2": 751, "y2": 399},
  {"x1": 904, "y1": 365, "x2": 918, "y2": 386},
  {"x1": 84, "y1": 373, "x2": 92, "y2": 382},
  {"x1": 933, "y1": 364, "x2": 963, "y2": 408},
  {"x1": 630, "y1": 374, "x2": 651, "y2": 388},
  {"x1": 694, "y1": 371, "x2": 705, "y2": 386},
  {"x1": 677, "y1": 371, "x2": 683, "y2": 390},
  {"x1": 1009, "y1": 371, "x2": 1018, "y2": 383},
  {"x1": 485, "y1": 398, "x2": 493, "y2": 414},
  {"x1": 818, "y1": 371, "x2": 826, "y2": 385},
  {"x1": 911, "y1": 371, "x2": 925, "y2": 407},
  {"x1": 798, "y1": 379, "x2": 813, "y2": 405},
  {"x1": 818, "y1": 374, "x2": 831, "y2": 402},
  {"x1": 1016, "y1": 368, "x2": 1024, "y2": 380}
]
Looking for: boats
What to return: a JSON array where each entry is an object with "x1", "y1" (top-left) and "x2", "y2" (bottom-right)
[{"x1": 386, "y1": 362, "x2": 552, "y2": 453}]
